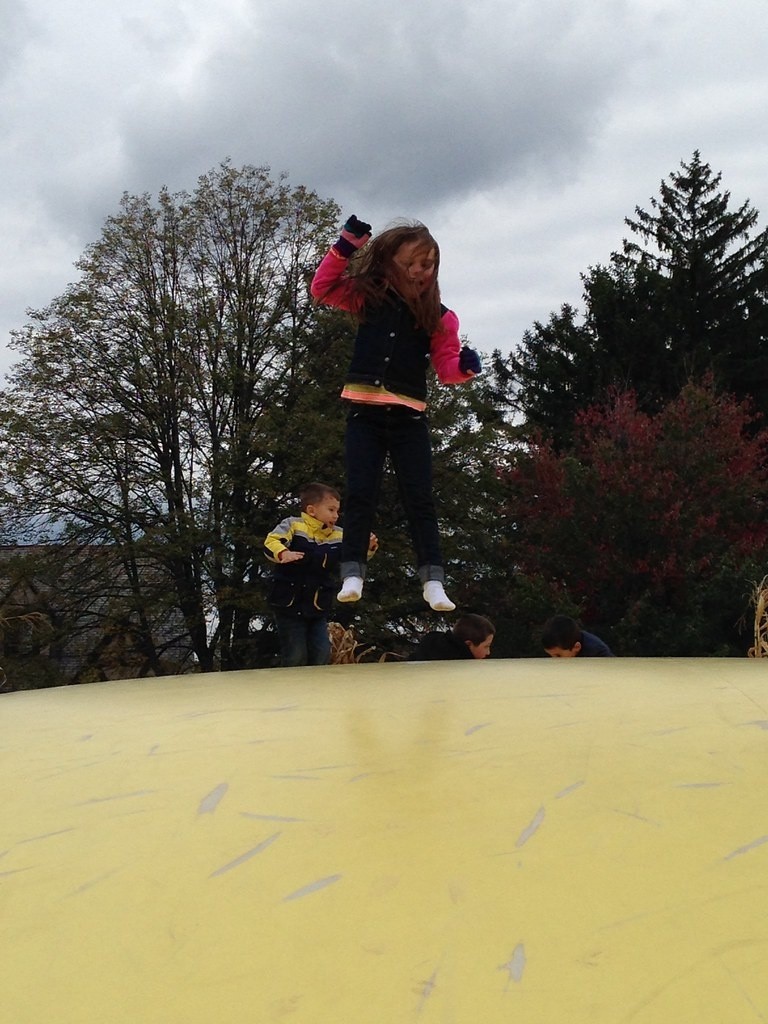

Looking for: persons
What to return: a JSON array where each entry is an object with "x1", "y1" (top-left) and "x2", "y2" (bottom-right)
[
  {"x1": 408, "y1": 613, "x2": 613, "y2": 662},
  {"x1": 310, "y1": 215, "x2": 482, "y2": 611},
  {"x1": 262, "y1": 483, "x2": 379, "y2": 667}
]
